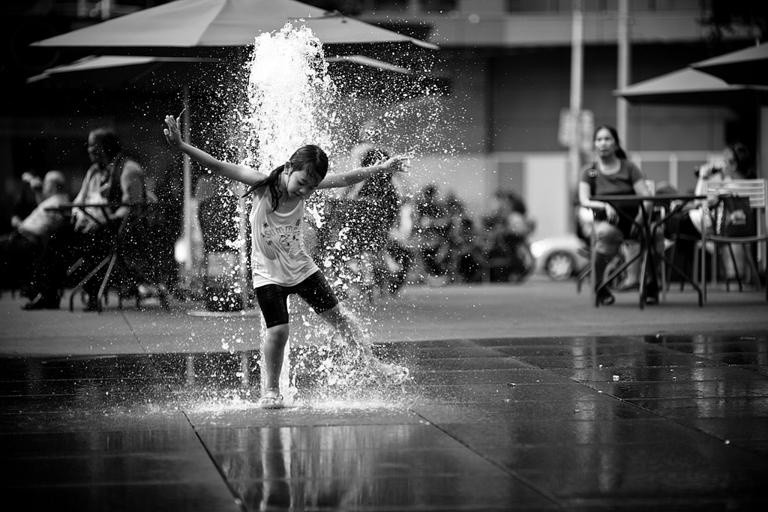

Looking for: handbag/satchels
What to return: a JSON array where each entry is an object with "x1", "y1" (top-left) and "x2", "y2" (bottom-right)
[{"x1": 715, "y1": 196, "x2": 752, "y2": 236}]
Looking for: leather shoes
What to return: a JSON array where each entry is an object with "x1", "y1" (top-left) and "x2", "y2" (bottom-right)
[{"x1": 23, "y1": 294, "x2": 59, "y2": 310}]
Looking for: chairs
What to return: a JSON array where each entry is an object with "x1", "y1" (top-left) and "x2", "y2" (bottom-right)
[
  {"x1": 43, "y1": 204, "x2": 169, "y2": 313},
  {"x1": 573, "y1": 178, "x2": 767, "y2": 305}
]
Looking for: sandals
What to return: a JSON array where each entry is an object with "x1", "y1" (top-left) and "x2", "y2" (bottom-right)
[
  {"x1": 644, "y1": 272, "x2": 658, "y2": 305},
  {"x1": 594, "y1": 280, "x2": 614, "y2": 305},
  {"x1": 260, "y1": 389, "x2": 284, "y2": 408},
  {"x1": 383, "y1": 365, "x2": 409, "y2": 387}
]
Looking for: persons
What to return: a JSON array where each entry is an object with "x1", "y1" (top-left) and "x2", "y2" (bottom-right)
[
  {"x1": 258, "y1": 429, "x2": 292, "y2": 509},
  {"x1": 163, "y1": 114, "x2": 414, "y2": 409},
  {"x1": 578, "y1": 125, "x2": 665, "y2": 306},
  {"x1": 410, "y1": 183, "x2": 536, "y2": 283},
  {"x1": 10, "y1": 127, "x2": 148, "y2": 313}
]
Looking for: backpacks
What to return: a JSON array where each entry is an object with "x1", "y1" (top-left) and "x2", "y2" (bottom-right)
[{"x1": 577, "y1": 167, "x2": 595, "y2": 245}]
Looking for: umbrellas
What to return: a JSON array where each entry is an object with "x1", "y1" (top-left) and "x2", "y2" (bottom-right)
[{"x1": 611, "y1": 41, "x2": 768, "y2": 272}]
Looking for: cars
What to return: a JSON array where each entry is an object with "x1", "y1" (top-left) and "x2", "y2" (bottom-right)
[{"x1": 530, "y1": 206, "x2": 753, "y2": 284}]
